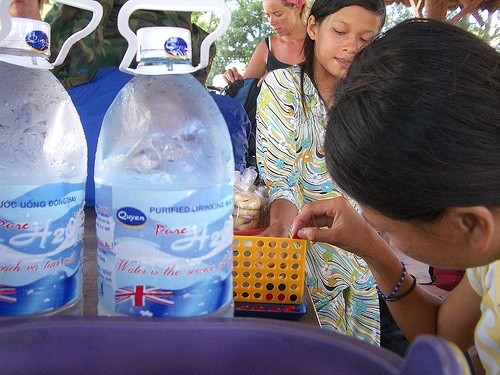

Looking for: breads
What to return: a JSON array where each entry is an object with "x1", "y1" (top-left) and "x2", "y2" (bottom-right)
[{"x1": 232, "y1": 191, "x2": 262, "y2": 230}]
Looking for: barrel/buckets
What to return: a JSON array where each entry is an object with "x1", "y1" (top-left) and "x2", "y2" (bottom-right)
[{"x1": 0, "y1": 311, "x2": 472, "y2": 375}]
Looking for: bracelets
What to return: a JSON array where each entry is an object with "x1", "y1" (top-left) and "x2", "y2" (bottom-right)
[
  {"x1": 383, "y1": 274, "x2": 416, "y2": 302},
  {"x1": 375, "y1": 261, "x2": 406, "y2": 296}
]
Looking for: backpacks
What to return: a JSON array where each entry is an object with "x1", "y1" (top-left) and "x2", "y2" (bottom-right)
[
  {"x1": 225, "y1": 77, "x2": 263, "y2": 156},
  {"x1": 43, "y1": 0, "x2": 194, "y2": 88}
]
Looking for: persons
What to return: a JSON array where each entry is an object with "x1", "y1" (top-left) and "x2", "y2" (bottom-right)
[
  {"x1": 223, "y1": 0, "x2": 311, "y2": 87},
  {"x1": 8, "y1": 0, "x2": 500, "y2": 375}
]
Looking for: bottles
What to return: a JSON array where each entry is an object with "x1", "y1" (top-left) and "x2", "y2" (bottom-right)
[
  {"x1": 0, "y1": 0, "x2": 105, "y2": 321},
  {"x1": 90, "y1": 1, "x2": 241, "y2": 319}
]
